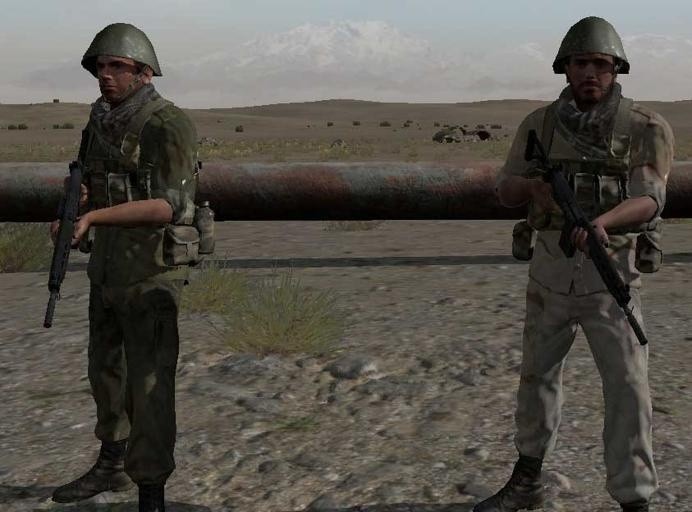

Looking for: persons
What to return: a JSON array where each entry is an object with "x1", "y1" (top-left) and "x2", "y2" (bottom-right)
[
  {"x1": 44, "y1": 23, "x2": 218, "y2": 510},
  {"x1": 473, "y1": 16, "x2": 674, "y2": 511}
]
